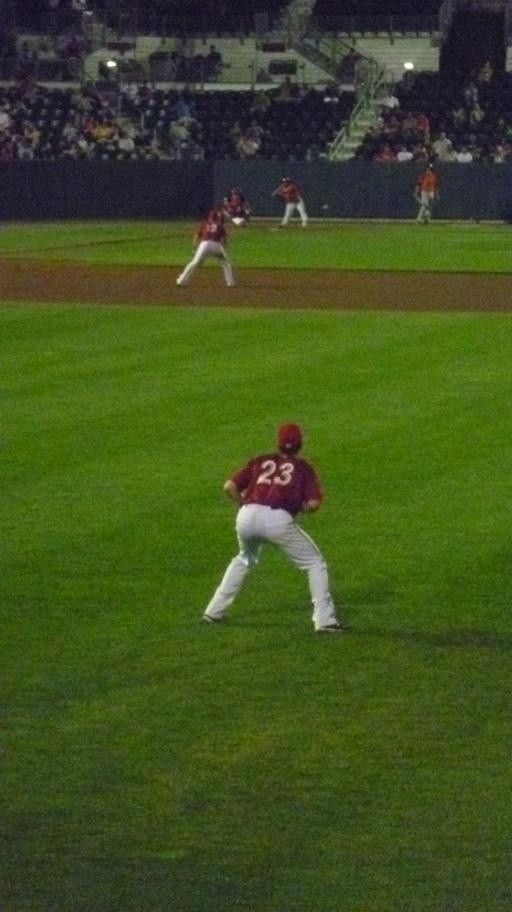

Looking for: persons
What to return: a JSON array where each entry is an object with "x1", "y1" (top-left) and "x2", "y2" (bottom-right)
[
  {"x1": 1, "y1": 39, "x2": 511, "y2": 165},
  {"x1": 174, "y1": 210, "x2": 237, "y2": 289},
  {"x1": 202, "y1": 420, "x2": 352, "y2": 635},
  {"x1": 271, "y1": 175, "x2": 310, "y2": 229},
  {"x1": 219, "y1": 186, "x2": 253, "y2": 229},
  {"x1": 414, "y1": 161, "x2": 440, "y2": 225}
]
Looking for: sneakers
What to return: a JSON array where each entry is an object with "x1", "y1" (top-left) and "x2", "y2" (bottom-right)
[
  {"x1": 317, "y1": 623, "x2": 350, "y2": 631},
  {"x1": 203, "y1": 614, "x2": 216, "y2": 624}
]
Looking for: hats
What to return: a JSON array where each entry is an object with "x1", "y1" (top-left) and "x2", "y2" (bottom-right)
[{"x1": 279, "y1": 423, "x2": 301, "y2": 448}]
